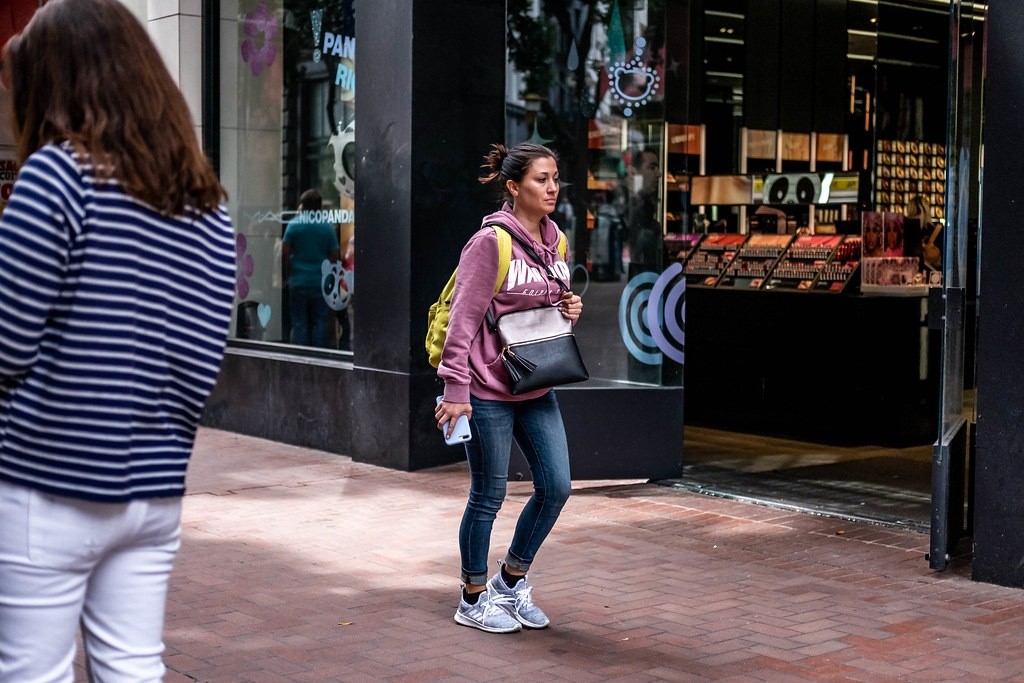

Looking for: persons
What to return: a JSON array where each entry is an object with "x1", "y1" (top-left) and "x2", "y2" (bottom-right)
[
  {"x1": 624, "y1": 147, "x2": 661, "y2": 262},
  {"x1": 283, "y1": 189, "x2": 353, "y2": 348},
  {"x1": 907, "y1": 196, "x2": 943, "y2": 264},
  {"x1": 434, "y1": 142, "x2": 583, "y2": 633},
  {"x1": 0, "y1": 0, "x2": 238, "y2": 683},
  {"x1": 863, "y1": 212, "x2": 903, "y2": 257}
]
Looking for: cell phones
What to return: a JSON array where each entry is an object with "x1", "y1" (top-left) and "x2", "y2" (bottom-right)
[{"x1": 436, "y1": 395, "x2": 472, "y2": 445}]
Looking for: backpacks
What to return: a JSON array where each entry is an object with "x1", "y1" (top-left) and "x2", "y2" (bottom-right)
[{"x1": 425, "y1": 225, "x2": 567, "y2": 368}]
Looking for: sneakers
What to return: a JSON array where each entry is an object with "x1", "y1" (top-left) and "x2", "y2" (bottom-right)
[
  {"x1": 486, "y1": 559, "x2": 550, "y2": 628},
  {"x1": 454, "y1": 584, "x2": 522, "y2": 632}
]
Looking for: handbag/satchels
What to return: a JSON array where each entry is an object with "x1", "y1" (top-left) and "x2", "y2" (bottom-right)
[
  {"x1": 922, "y1": 224, "x2": 941, "y2": 266},
  {"x1": 482, "y1": 221, "x2": 589, "y2": 395}
]
